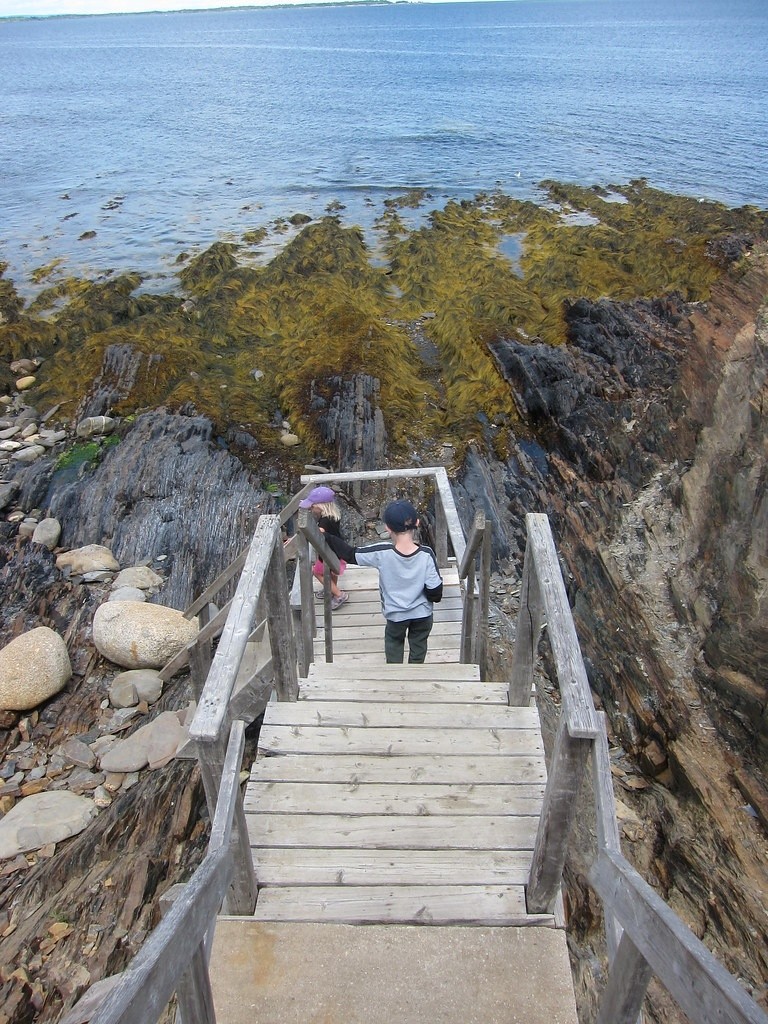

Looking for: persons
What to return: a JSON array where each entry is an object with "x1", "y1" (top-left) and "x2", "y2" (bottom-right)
[
  {"x1": 318, "y1": 498, "x2": 444, "y2": 664},
  {"x1": 282, "y1": 486, "x2": 349, "y2": 610}
]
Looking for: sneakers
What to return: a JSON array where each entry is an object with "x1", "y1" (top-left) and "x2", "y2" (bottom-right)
[
  {"x1": 316, "y1": 590, "x2": 324, "y2": 599},
  {"x1": 332, "y1": 593, "x2": 349, "y2": 610}
]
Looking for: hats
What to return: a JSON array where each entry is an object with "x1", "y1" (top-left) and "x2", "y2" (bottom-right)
[
  {"x1": 299, "y1": 487, "x2": 335, "y2": 509},
  {"x1": 383, "y1": 500, "x2": 417, "y2": 531}
]
